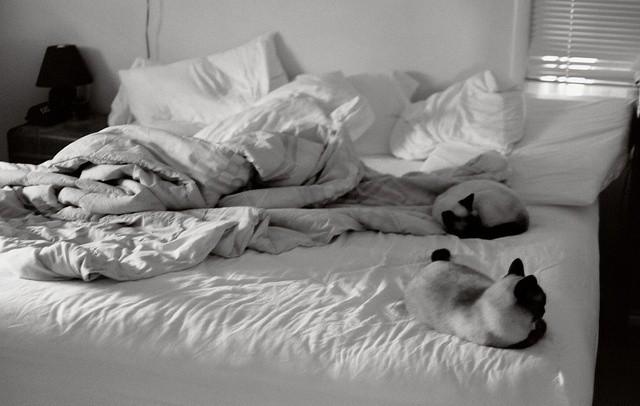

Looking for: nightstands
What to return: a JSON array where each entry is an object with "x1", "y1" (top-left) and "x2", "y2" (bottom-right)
[{"x1": 7, "y1": 113, "x2": 109, "y2": 165}]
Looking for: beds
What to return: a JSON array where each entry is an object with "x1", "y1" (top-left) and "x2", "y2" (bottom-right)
[{"x1": 0, "y1": 32, "x2": 640, "y2": 406}]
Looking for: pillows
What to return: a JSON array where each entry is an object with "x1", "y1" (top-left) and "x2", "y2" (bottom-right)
[
  {"x1": 118, "y1": 32, "x2": 289, "y2": 127},
  {"x1": 107, "y1": 57, "x2": 163, "y2": 126},
  {"x1": 508, "y1": 92, "x2": 637, "y2": 206}
]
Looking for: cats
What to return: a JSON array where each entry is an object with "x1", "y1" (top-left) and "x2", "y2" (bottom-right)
[
  {"x1": 404, "y1": 248, "x2": 547, "y2": 350},
  {"x1": 431, "y1": 180, "x2": 529, "y2": 239}
]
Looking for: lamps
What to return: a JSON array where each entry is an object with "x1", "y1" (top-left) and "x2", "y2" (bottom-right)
[{"x1": 35, "y1": 44, "x2": 94, "y2": 114}]
